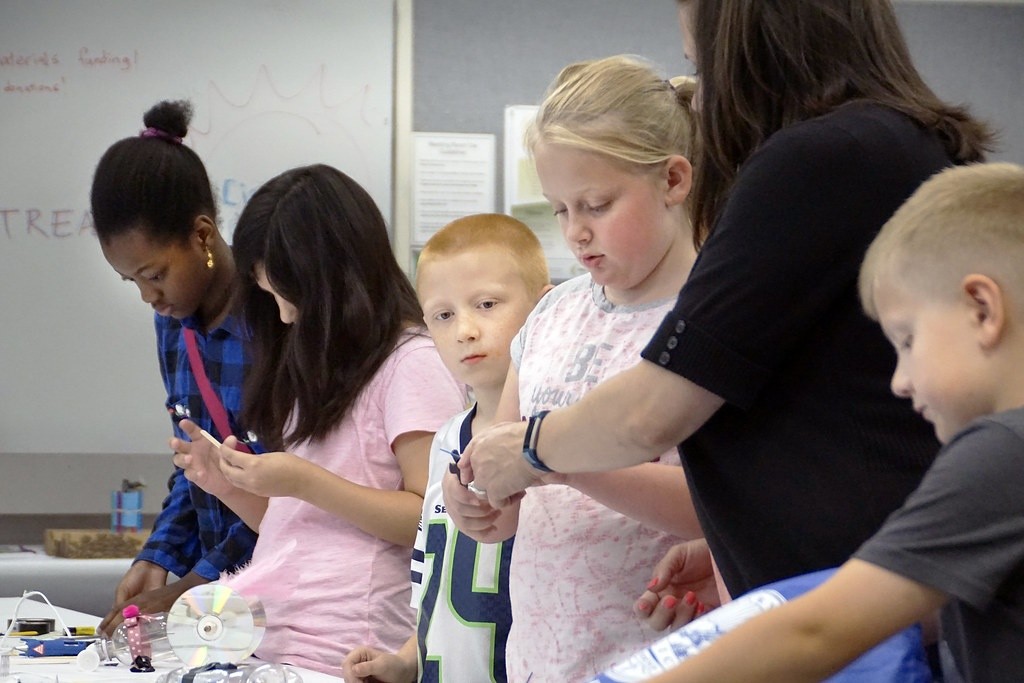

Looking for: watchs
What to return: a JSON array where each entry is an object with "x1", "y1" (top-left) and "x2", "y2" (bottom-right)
[{"x1": 522, "y1": 409, "x2": 554, "y2": 472}]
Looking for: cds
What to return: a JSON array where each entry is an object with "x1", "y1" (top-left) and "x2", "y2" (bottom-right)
[{"x1": 165, "y1": 581, "x2": 266, "y2": 668}]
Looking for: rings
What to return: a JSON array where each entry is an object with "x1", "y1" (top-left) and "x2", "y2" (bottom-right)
[{"x1": 467, "y1": 480, "x2": 485, "y2": 495}]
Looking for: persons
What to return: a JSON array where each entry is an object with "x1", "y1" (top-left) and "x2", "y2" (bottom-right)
[
  {"x1": 90, "y1": 99, "x2": 269, "y2": 642},
  {"x1": 170, "y1": 163, "x2": 471, "y2": 683},
  {"x1": 641, "y1": 162, "x2": 1024, "y2": 683},
  {"x1": 458, "y1": 0, "x2": 998, "y2": 683},
  {"x1": 342, "y1": 214, "x2": 555, "y2": 683},
  {"x1": 443, "y1": 55, "x2": 714, "y2": 683}
]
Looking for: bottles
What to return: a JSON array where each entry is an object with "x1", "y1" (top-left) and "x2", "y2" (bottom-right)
[{"x1": 95, "y1": 611, "x2": 175, "y2": 666}]
